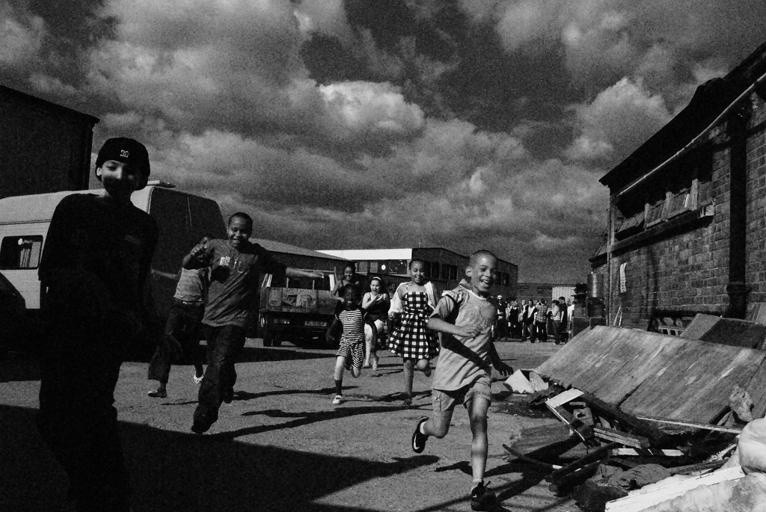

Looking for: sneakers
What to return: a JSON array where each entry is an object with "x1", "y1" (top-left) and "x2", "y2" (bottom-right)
[
  {"x1": 470, "y1": 480, "x2": 510, "y2": 512},
  {"x1": 194, "y1": 373, "x2": 204, "y2": 384},
  {"x1": 332, "y1": 394, "x2": 344, "y2": 405},
  {"x1": 147, "y1": 389, "x2": 167, "y2": 398},
  {"x1": 412, "y1": 415, "x2": 429, "y2": 454}
]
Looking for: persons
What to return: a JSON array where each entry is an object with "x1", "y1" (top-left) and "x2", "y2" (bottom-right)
[
  {"x1": 181, "y1": 211, "x2": 325, "y2": 433},
  {"x1": 330, "y1": 258, "x2": 443, "y2": 409},
  {"x1": 36, "y1": 136, "x2": 180, "y2": 512},
  {"x1": 147, "y1": 231, "x2": 217, "y2": 398},
  {"x1": 492, "y1": 294, "x2": 569, "y2": 344},
  {"x1": 411, "y1": 249, "x2": 514, "y2": 512}
]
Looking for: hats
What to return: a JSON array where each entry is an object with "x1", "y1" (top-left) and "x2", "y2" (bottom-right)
[{"x1": 96, "y1": 137, "x2": 150, "y2": 175}]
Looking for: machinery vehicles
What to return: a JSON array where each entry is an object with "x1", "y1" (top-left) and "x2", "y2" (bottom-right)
[{"x1": 257, "y1": 267, "x2": 337, "y2": 349}]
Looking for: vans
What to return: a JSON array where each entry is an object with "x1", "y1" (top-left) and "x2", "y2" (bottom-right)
[{"x1": 0, "y1": 186, "x2": 228, "y2": 342}]
